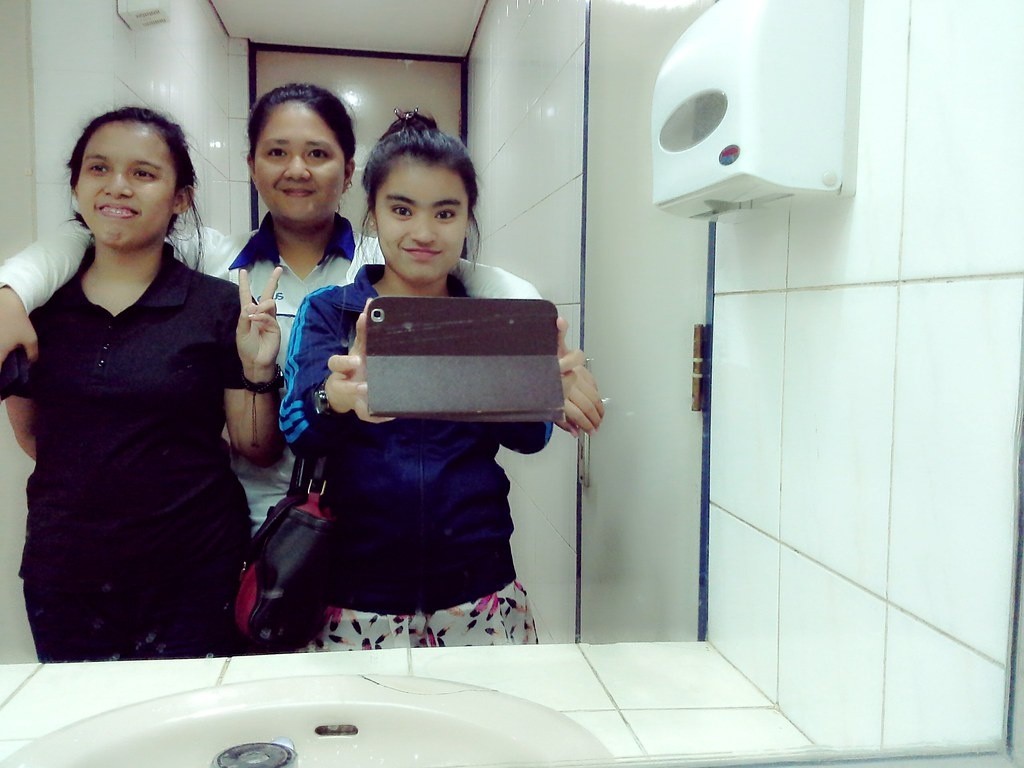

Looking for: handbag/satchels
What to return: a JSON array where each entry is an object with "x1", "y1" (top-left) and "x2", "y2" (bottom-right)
[{"x1": 225, "y1": 454, "x2": 341, "y2": 652}]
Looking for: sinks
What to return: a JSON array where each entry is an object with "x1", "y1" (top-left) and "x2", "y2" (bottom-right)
[{"x1": 0, "y1": 673, "x2": 619, "y2": 768}]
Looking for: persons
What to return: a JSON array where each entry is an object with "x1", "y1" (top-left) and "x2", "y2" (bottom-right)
[
  {"x1": 0, "y1": 104, "x2": 284, "y2": 663},
  {"x1": 0, "y1": 83, "x2": 605, "y2": 525},
  {"x1": 276, "y1": 107, "x2": 606, "y2": 656}
]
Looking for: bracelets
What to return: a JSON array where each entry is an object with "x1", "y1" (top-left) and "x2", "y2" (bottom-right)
[{"x1": 239, "y1": 364, "x2": 285, "y2": 394}]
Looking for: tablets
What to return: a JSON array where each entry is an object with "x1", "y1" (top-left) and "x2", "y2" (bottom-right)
[{"x1": 364, "y1": 296, "x2": 567, "y2": 423}]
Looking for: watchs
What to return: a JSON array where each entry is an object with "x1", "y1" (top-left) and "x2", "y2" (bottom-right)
[{"x1": 314, "y1": 378, "x2": 337, "y2": 417}]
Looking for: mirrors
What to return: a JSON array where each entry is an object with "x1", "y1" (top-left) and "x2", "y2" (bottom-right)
[{"x1": 0, "y1": 0, "x2": 1018, "y2": 768}]
